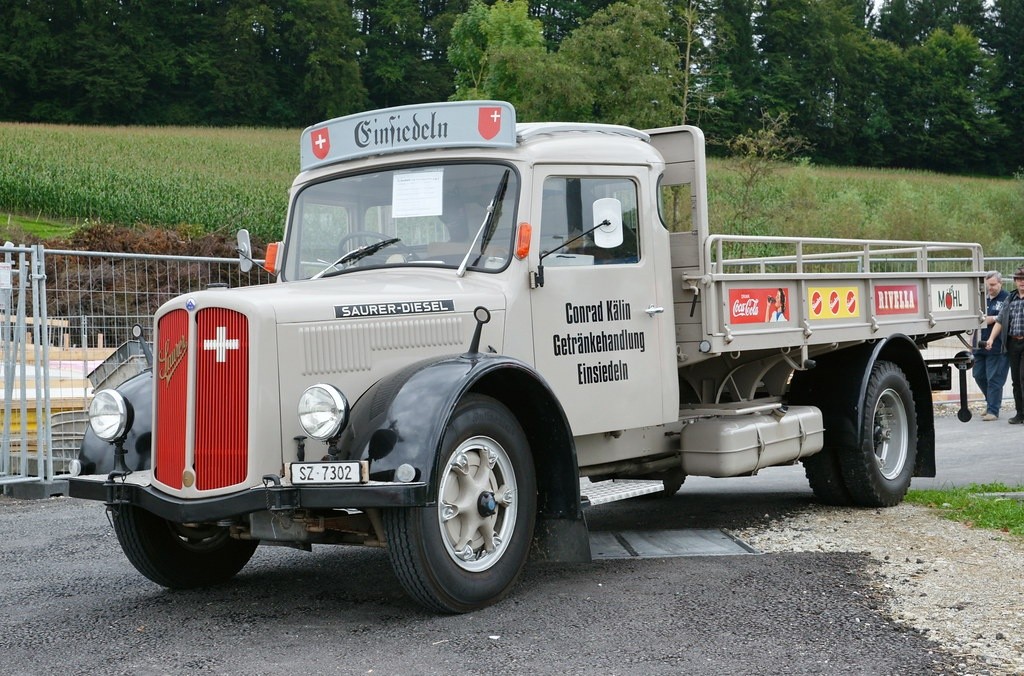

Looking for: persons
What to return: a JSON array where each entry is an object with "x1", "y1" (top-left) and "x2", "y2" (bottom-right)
[
  {"x1": 972, "y1": 268, "x2": 1024, "y2": 423},
  {"x1": 764, "y1": 288, "x2": 788, "y2": 323},
  {"x1": 539, "y1": 192, "x2": 596, "y2": 257}
]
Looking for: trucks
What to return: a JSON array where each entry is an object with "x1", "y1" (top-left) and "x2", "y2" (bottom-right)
[{"x1": 68, "y1": 101, "x2": 992, "y2": 618}]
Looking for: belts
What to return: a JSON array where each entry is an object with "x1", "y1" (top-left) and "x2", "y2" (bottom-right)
[{"x1": 1012, "y1": 336, "x2": 1024, "y2": 340}]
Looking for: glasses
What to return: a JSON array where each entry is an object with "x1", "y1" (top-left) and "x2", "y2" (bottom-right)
[{"x1": 1015, "y1": 277, "x2": 1024, "y2": 281}]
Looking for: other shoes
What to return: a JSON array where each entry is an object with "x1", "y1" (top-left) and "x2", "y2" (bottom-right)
[
  {"x1": 981, "y1": 409, "x2": 998, "y2": 421},
  {"x1": 1008, "y1": 413, "x2": 1024, "y2": 424}
]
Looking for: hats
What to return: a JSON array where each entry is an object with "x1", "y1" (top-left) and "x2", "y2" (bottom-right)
[{"x1": 1012, "y1": 266, "x2": 1024, "y2": 277}]
